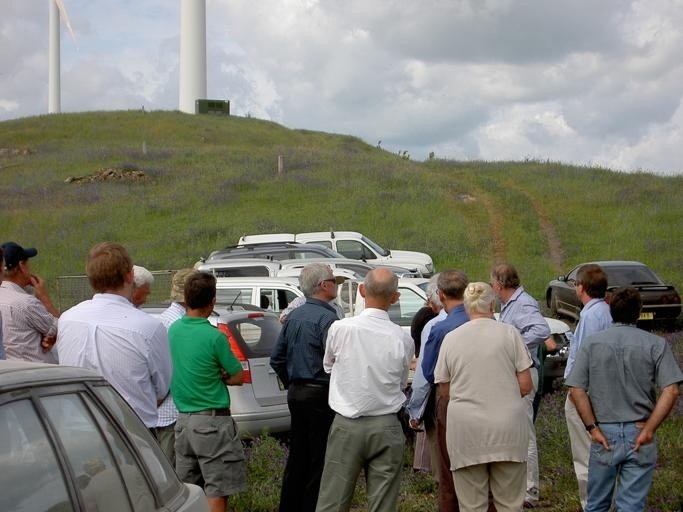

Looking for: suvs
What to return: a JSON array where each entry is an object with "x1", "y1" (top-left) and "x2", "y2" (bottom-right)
[
  {"x1": 193, "y1": 281, "x2": 309, "y2": 313},
  {"x1": 197, "y1": 257, "x2": 378, "y2": 310},
  {"x1": 237, "y1": 232, "x2": 435, "y2": 277},
  {"x1": 204, "y1": 241, "x2": 423, "y2": 279}
]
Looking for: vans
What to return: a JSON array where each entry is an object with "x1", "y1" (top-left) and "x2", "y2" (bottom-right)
[{"x1": 137, "y1": 306, "x2": 292, "y2": 438}]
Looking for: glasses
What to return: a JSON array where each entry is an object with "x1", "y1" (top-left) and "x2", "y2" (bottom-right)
[
  {"x1": 435, "y1": 289, "x2": 446, "y2": 299},
  {"x1": 317, "y1": 277, "x2": 337, "y2": 286}
]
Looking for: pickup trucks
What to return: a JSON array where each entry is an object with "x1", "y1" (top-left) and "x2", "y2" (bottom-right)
[{"x1": 352, "y1": 270, "x2": 573, "y2": 393}]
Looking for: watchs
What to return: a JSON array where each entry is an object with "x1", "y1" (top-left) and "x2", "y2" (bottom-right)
[{"x1": 585, "y1": 420, "x2": 601, "y2": 433}]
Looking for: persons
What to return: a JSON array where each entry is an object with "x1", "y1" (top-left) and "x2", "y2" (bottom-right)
[
  {"x1": 409, "y1": 272, "x2": 447, "y2": 491},
  {"x1": 0, "y1": 246, "x2": 5, "y2": 361},
  {"x1": 1, "y1": 241, "x2": 61, "y2": 365},
  {"x1": 532, "y1": 333, "x2": 557, "y2": 424},
  {"x1": 271, "y1": 264, "x2": 341, "y2": 512},
  {"x1": 279, "y1": 263, "x2": 347, "y2": 323},
  {"x1": 129, "y1": 264, "x2": 153, "y2": 309},
  {"x1": 167, "y1": 272, "x2": 242, "y2": 512},
  {"x1": 316, "y1": 268, "x2": 416, "y2": 512},
  {"x1": 56, "y1": 242, "x2": 170, "y2": 443},
  {"x1": 412, "y1": 274, "x2": 443, "y2": 474},
  {"x1": 155, "y1": 269, "x2": 199, "y2": 470},
  {"x1": 563, "y1": 287, "x2": 683, "y2": 511},
  {"x1": 491, "y1": 264, "x2": 549, "y2": 508},
  {"x1": 564, "y1": 264, "x2": 614, "y2": 511},
  {"x1": 422, "y1": 272, "x2": 500, "y2": 512},
  {"x1": 434, "y1": 280, "x2": 532, "y2": 511}
]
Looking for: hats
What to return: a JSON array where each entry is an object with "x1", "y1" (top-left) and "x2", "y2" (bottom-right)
[
  {"x1": 170, "y1": 268, "x2": 200, "y2": 303},
  {"x1": 0, "y1": 242, "x2": 38, "y2": 271}
]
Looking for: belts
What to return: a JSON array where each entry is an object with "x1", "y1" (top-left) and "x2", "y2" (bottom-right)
[
  {"x1": 180, "y1": 408, "x2": 232, "y2": 417},
  {"x1": 598, "y1": 418, "x2": 646, "y2": 424},
  {"x1": 293, "y1": 379, "x2": 328, "y2": 389}
]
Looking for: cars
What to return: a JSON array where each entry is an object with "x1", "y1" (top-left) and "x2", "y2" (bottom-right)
[
  {"x1": 1, "y1": 359, "x2": 215, "y2": 512},
  {"x1": 545, "y1": 261, "x2": 682, "y2": 329}
]
